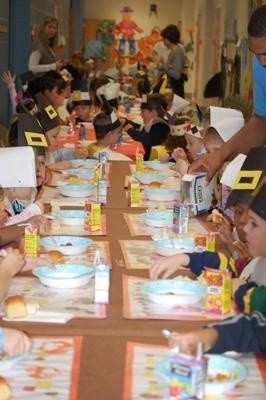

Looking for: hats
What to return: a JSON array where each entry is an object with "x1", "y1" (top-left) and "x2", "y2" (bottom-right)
[
  {"x1": 0, "y1": 146, "x2": 37, "y2": 189},
  {"x1": 95, "y1": 83, "x2": 121, "y2": 103}
]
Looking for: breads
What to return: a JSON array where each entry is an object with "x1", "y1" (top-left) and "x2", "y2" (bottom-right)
[
  {"x1": 0, "y1": 376, "x2": 11, "y2": 400},
  {"x1": 46, "y1": 249, "x2": 64, "y2": 264},
  {"x1": 4, "y1": 296, "x2": 26, "y2": 319},
  {"x1": 147, "y1": 181, "x2": 161, "y2": 190},
  {"x1": 67, "y1": 174, "x2": 79, "y2": 184}
]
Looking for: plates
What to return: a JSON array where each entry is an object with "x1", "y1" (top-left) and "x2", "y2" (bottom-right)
[
  {"x1": 32, "y1": 157, "x2": 101, "y2": 290},
  {"x1": 137, "y1": 163, "x2": 207, "y2": 306},
  {"x1": 156, "y1": 352, "x2": 247, "y2": 393},
  {"x1": 0, "y1": 352, "x2": 29, "y2": 371}
]
